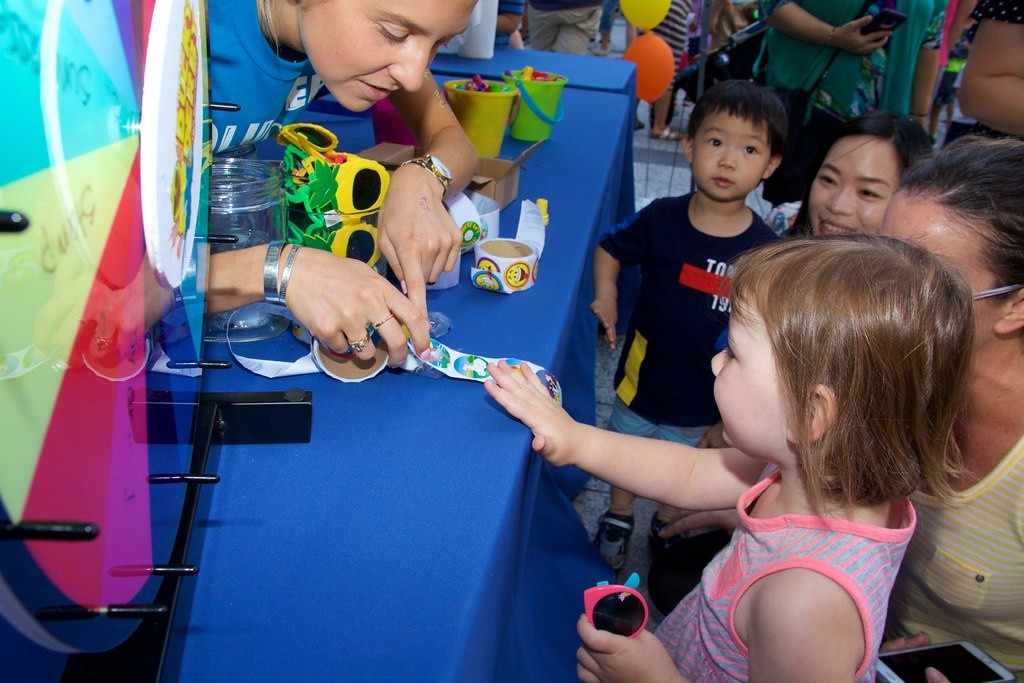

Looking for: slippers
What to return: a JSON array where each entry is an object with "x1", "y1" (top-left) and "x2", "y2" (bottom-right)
[{"x1": 649, "y1": 126, "x2": 682, "y2": 141}]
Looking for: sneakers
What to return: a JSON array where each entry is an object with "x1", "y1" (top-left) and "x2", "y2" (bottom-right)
[
  {"x1": 593, "y1": 510, "x2": 635, "y2": 572},
  {"x1": 648, "y1": 511, "x2": 687, "y2": 557}
]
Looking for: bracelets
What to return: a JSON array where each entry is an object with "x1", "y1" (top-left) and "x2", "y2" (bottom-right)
[{"x1": 264, "y1": 240, "x2": 303, "y2": 305}]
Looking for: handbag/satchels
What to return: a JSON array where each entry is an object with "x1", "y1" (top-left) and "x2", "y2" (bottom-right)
[{"x1": 751, "y1": 76, "x2": 811, "y2": 155}]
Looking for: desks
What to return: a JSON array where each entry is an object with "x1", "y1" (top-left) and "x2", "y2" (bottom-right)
[
  {"x1": 0, "y1": 74, "x2": 640, "y2": 683},
  {"x1": 434, "y1": 39, "x2": 636, "y2": 91}
]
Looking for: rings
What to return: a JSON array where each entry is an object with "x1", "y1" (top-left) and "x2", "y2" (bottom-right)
[
  {"x1": 347, "y1": 333, "x2": 370, "y2": 352},
  {"x1": 372, "y1": 314, "x2": 394, "y2": 331}
]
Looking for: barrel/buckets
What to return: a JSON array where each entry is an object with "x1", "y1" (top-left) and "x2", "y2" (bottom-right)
[
  {"x1": 502, "y1": 70, "x2": 569, "y2": 141},
  {"x1": 443, "y1": 80, "x2": 520, "y2": 159}
]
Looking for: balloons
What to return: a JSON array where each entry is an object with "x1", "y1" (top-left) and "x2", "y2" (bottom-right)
[
  {"x1": 624, "y1": 31, "x2": 675, "y2": 108},
  {"x1": 619, "y1": 0, "x2": 671, "y2": 32}
]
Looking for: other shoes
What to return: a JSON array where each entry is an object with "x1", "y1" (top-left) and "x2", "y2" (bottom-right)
[
  {"x1": 634, "y1": 122, "x2": 645, "y2": 131},
  {"x1": 928, "y1": 134, "x2": 936, "y2": 147},
  {"x1": 593, "y1": 43, "x2": 611, "y2": 55}
]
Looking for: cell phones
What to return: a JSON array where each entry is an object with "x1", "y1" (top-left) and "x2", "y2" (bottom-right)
[
  {"x1": 873, "y1": 639, "x2": 1016, "y2": 683},
  {"x1": 861, "y1": 9, "x2": 906, "y2": 41}
]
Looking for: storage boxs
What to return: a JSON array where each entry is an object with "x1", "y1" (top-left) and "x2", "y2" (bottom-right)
[{"x1": 354, "y1": 138, "x2": 545, "y2": 213}]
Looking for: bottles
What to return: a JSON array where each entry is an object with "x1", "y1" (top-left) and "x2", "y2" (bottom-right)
[{"x1": 203, "y1": 159, "x2": 289, "y2": 343}]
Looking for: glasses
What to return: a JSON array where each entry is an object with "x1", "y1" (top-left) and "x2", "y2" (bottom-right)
[{"x1": 584, "y1": 572, "x2": 649, "y2": 639}]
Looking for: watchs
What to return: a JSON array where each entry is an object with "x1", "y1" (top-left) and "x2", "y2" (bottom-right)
[{"x1": 401, "y1": 154, "x2": 452, "y2": 196}]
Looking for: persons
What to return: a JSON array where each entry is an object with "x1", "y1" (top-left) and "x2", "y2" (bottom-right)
[
  {"x1": 484, "y1": 233, "x2": 973, "y2": 683},
  {"x1": 646, "y1": 136, "x2": 1024, "y2": 683},
  {"x1": 207, "y1": 0, "x2": 479, "y2": 368},
  {"x1": 590, "y1": 79, "x2": 783, "y2": 569},
  {"x1": 494, "y1": 0, "x2": 1024, "y2": 240}
]
